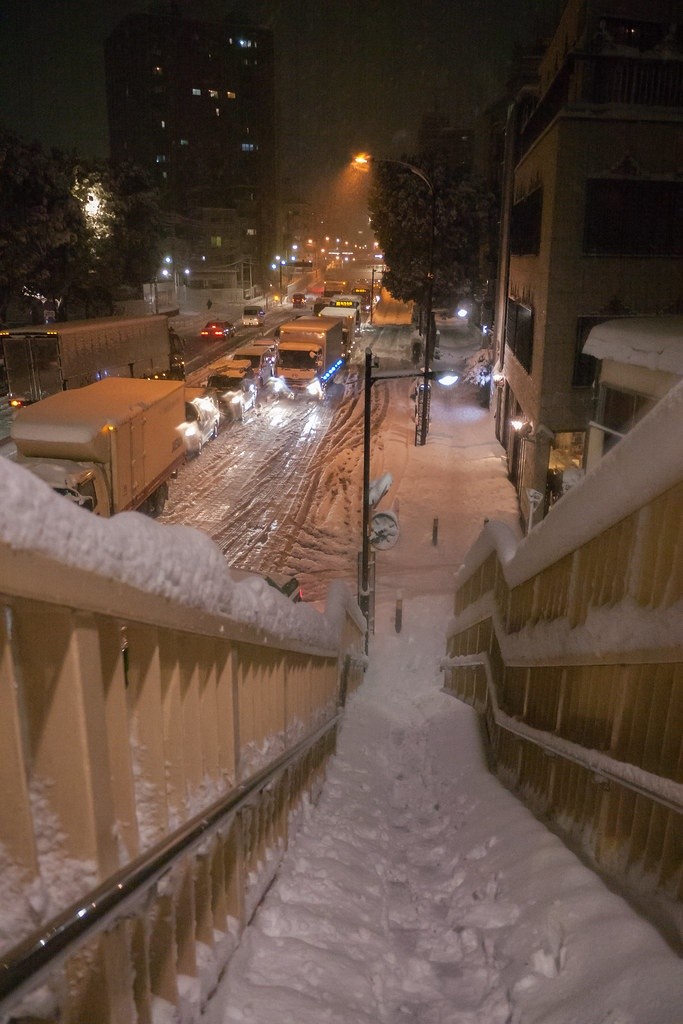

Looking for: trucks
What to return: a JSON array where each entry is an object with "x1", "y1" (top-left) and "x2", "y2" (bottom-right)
[
  {"x1": 9, "y1": 376, "x2": 189, "y2": 519},
  {"x1": 274, "y1": 315, "x2": 346, "y2": 399},
  {"x1": 323, "y1": 280, "x2": 350, "y2": 297},
  {"x1": 318, "y1": 306, "x2": 357, "y2": 363}
]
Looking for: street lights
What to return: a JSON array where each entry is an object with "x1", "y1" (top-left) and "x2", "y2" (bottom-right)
[
  {"x1": 354, "y1": 153, "x2": 439, "y2": 447},
  {"x1": 358, "y1": 344, "x2": 460, "y2": 638}
]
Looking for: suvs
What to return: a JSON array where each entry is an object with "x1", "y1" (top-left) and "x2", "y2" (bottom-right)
[{"x1": 293, "y1": 293, "x2": 306, "y2": 307}]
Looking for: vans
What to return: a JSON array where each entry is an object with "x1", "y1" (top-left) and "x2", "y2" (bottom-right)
[
  {"x1": 243, "y1": 306, "x2": 265, "y2": 326},
  {"x1": 183, "y1": 386, "x2": 220, "y2": 455},
  {"x1": 314, "y1": 297, "x2": 331, "y2": 316},
  {"x1": 233, "y1": 347, "x2": 273, "y2": 389},
  {"x1": 207, "y1": 360, "x2": 258, "y2": 421}
]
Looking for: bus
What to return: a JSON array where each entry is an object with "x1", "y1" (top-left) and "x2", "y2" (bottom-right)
[
  {"x1": 330, "y1": 294, "x2": 362, "y2": 334},
  {"x1": 352, "y1": 284, "x2": 376, "y2": 312}
]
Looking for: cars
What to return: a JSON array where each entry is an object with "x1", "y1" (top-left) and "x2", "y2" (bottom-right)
[
  {"x1": 254, "y1": 341, "x2": 279, "y2": 360},
  {"x1": 200, "y1": 319, "x2": 236, "y2": 341}
]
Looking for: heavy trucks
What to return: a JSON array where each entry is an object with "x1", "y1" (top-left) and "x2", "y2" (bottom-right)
[{"x1": 0, "y1": 311, "x2": 186, "y2": 412}]
